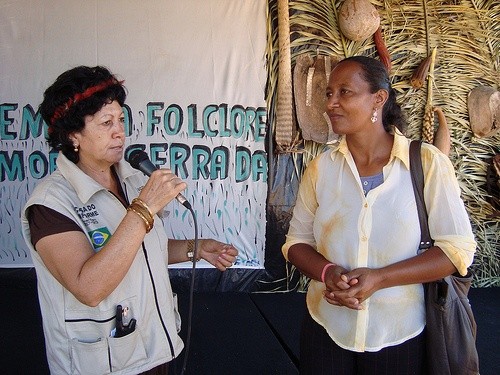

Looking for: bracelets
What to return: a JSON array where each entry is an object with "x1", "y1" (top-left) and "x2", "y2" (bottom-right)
[
  {"x1": 127, "y1": 198, "x2": 155, "y2": 233},
  {"x1": 321, "y1": 263, "x2": 335, "y2": 284}
]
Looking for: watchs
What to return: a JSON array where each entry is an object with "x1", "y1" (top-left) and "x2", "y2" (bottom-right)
[{"x1": 186, "y1": 240, "x2": 200, "y2": 262}]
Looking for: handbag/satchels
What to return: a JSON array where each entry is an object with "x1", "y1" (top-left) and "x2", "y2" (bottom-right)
[{"x1": 422, "y1": 269, "x2": 480, "y2": 375}]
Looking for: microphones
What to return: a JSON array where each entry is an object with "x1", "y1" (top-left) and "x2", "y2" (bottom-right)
[{"x1": 129, "y1": 149, "x2": 192, "y2": 210}]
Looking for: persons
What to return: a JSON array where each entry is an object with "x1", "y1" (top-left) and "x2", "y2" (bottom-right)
[
  {"x1": 282, "y1": 56, "x2": 483, "y2": 375},
  {"x1": 21, "y1": 64, "x2": 238, "y2": 375}
]
